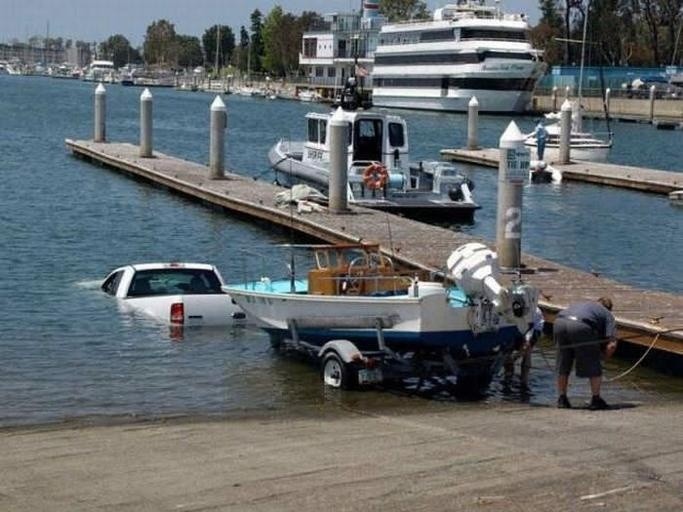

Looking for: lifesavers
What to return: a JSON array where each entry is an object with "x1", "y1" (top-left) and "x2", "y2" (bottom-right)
[{"x1": 362, "y1": 164, "x2": 388, "y2": 191}]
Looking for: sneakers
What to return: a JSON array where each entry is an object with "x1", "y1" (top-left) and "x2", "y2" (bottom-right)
[
  {"x1": 557, "y1": 396, "x2": 572, "y2": 408},
  {"x1": 587, "y1": 397, "x2": 611, "y2": 411}
]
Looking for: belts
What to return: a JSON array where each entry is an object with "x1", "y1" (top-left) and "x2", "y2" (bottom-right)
[{"x1": 557, "y1": 314, "x2": 590, "y2": 325}]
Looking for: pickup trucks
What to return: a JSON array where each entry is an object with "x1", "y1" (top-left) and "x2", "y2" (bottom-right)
[{"x1": 101, "y1": 263, "x2": 247, "y2": 326}]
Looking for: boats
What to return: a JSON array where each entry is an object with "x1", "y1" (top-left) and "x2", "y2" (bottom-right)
[
  {"x1": 527, "y1": 102, "x2": 612, "y2": 161},
  {"x1": 298, "y1": 0, "x2": 548, "y2": 113},
  {"x1": 220, "y1": 245, "x2": 539, "y2": 355},
  {"x1": 269, "y1": 112, "x2": 482, "y2": 223}
]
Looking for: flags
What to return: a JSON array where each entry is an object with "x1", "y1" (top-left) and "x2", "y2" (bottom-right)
[{"x1": 353, "y1": 63, "x2": 371, "y2": 79}]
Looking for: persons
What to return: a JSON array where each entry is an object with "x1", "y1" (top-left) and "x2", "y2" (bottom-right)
[
  {"x1": 531, "y1": 117, "x2": 550, "y2": 163},
  {"x1": 552, "y1": 295, "x2": 618, "y2": 410},
  {"x1": 497, "y1": 298, "x2": 546, "y2": 393}
]
